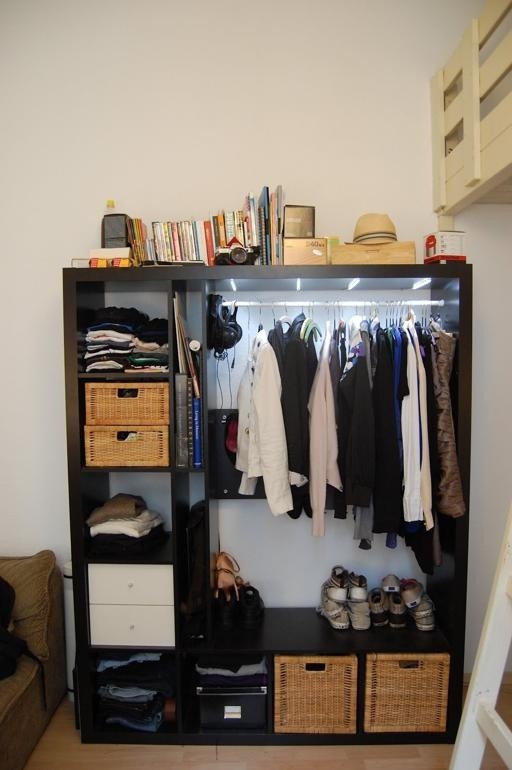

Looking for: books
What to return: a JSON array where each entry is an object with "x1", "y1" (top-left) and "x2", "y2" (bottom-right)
[{"x1": 127, "y1": 185, "x2": 283, "y2": 267}]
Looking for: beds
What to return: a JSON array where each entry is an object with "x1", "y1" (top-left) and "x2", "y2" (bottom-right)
[{"x1": 429, "y1": 0, "x2": 512, "y2": 232}]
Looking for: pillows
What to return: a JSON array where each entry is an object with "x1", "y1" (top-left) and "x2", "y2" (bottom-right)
[{"x1": 0, "y1": 547, "x2": 57, "y2": 664}]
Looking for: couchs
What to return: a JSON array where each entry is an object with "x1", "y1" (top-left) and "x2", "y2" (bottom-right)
[{"x1": 0, "y1": 564, "x2": 68, "y2": 770}]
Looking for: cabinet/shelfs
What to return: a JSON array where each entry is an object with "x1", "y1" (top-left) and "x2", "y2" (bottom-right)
[{"x1": 60, "y1": 257, "x2": 474, "y2": 750}]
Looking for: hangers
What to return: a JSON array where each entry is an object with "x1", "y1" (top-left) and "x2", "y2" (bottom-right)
[{"x1": 245, "y1": 297, "x2": 447, "y2": 337}]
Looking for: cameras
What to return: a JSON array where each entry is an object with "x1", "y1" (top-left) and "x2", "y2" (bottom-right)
[{"x1": 218, "y1": 245, "x2": 253, "y2": 265}]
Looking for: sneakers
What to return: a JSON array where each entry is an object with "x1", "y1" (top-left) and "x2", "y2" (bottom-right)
[
  {"x1": 315, "y1": 565, "x2": 436, "y2": 633},
  {"x1": 215, "y1": 585, "x2": 264, "y2": 633}
]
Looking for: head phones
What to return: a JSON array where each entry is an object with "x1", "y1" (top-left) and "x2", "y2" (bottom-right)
[{"x1": 209, "y1": 294, "x2": 241, "y2": 353}]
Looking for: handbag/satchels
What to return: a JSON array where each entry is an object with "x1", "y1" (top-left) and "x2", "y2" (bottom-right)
[{"x1": 217, "y1": 551, "x2": 238, "y2": 588}]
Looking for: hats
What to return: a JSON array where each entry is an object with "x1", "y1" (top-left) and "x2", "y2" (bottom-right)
[{"x1": 344, "y1": 213, "x2": 398, "y2": 245}]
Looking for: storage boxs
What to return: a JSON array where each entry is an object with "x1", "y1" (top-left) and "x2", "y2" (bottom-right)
[
  {"x1": 282, "y1": 204, "x2": 415, "y2": 265},
  {"x1": 196, "y1": 686, "x2": 267, "y2": 730}
]
